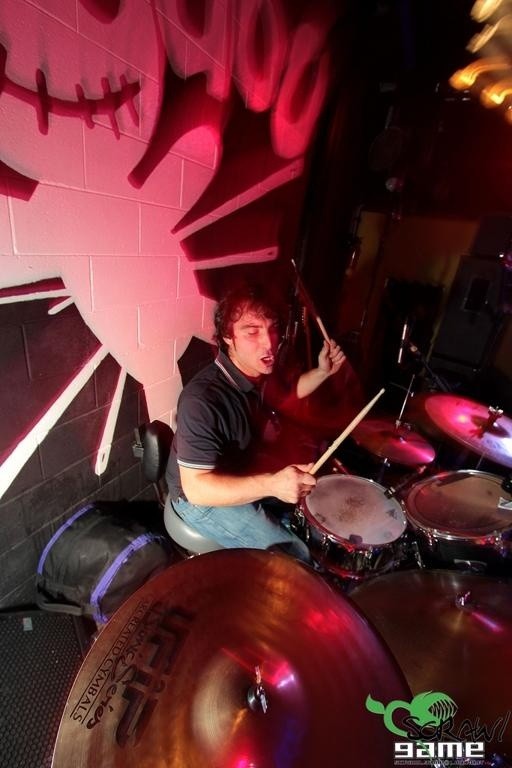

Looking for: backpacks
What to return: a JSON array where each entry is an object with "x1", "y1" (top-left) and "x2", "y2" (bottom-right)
[{"x1": 33, "y1": 502, "x2": 176, "y2": 626}]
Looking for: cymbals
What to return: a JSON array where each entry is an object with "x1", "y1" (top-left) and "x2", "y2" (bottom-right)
[
  {"x1": 347, "y1": 570, "x2": 512, "y2": 763},
  {"x1": 52, "y1": 550, "x2": 420, "y2": 763},
  {"x1": 425, "y1": 395, "x2": 512, "y2": 465},
  {"x1": 352, "y1": 419, "x2": 436, "y2": 468}
]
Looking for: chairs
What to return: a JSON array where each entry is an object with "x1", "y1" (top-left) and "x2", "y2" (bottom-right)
[{"x1": 144, "y1": 419, "x2": 226, "y2": 561}]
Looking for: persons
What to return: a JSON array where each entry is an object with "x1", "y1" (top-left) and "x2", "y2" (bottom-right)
[{"x1": 166, "y1": 283, "x2": 349, "y2": 571}]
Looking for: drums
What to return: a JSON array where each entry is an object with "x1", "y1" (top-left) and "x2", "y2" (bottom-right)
[
  {"x1": 295, "y1": 474, "x2": 410, "y2": 581},
  {"x1": 403, "y1": 470, "x2": 512, "y2": 579}
]
